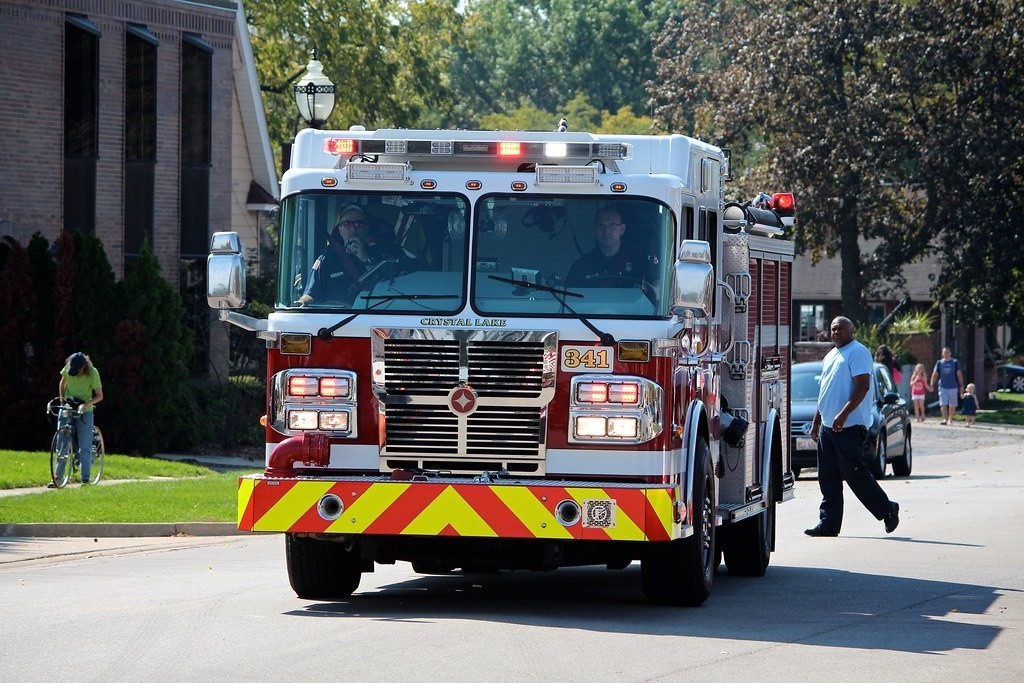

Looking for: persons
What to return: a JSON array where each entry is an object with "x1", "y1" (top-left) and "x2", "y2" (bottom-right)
[
  {"x1": 874, "y1": 345, "x2": 903, "y2": 373},
  {"x1": 817, "y1": 331, "x2": 828, "y2": 342},
  {"x1": 930, "y1": 346, "x2": 964, "y2": 424},
  {"x1": 803, "y1": 316, "x2": 900, "y2": 537},
  {"x1": 960, "y1": 383, "x2": 979, "y2": 427},
  {"x1": 910, "y1": 364, "x2": 929, "y2": 422},
  {"x1": 47, "y1": 352, "x2": 103, "y2": 488},
  {"x1": 565, "y1": 206, "x2": 660, "y2": 288},
  {"x1": 294, "y1": 210, "x2": 412, "y2": 307}
]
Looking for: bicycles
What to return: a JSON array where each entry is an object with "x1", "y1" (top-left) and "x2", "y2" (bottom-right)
[{"x1": 45, "y1": 396, "x2": 106, "y2": 489}]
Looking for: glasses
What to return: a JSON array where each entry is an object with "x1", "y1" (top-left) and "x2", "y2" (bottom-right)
[
  {"x1": 595, "y1": 223, "x2": 623, "y2": 230},
  {"x1": 339, "y1": 220, "x2": 366, "y2": 228}
]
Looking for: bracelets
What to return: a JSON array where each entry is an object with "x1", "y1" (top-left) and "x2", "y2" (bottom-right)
[{"x1": 365, "y1": 258, "x2": 375, "y2": 264}]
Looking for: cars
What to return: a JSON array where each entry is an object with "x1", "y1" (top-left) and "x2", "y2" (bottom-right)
[
  {"x1": 790, "y1": 359, "x2": 913, "y2": 483},
  {"x1": 995, "y1": 363, "x2": 1024, "y2": 394}
]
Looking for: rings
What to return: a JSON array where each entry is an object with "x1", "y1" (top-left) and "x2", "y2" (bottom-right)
[{"x1": 838, "y1": 426, "x2": 840, "y2": 427}]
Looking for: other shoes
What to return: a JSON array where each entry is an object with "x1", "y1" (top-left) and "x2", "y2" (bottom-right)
[
  {"x1": 940, "y1": 419, "x2": 947, "y2": 425},
  {"x1": 965, "y1": 423, "x2": 970, "y2": 427},
  {"x1": 947, "y1": 420, "x2": 953, "y2": 426},
  {"x1": 916, "y1": 417, "x2": 920, "y2": 422},
  {"x1": 921, "y1": 417, "x2": 926, "y2": 421},
  {"x1": 971, "y1": 422, "x2": 976, "y2": 425}
]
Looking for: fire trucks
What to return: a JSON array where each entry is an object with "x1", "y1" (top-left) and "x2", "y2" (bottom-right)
[{"x1": 202, "y1": 125, "x2": 799, "y2": 611}]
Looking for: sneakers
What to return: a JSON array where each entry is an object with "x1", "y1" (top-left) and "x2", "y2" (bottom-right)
[
  {"x1": 79, "y1": 482, "x2": 91, "y2": 487},
  {"x1": 804, "y1": 526, "x2": 839, "y2": 537},
  {"x1": 47, "y1": 478, "x2": 62, "y2": 488},
  {"x1": 883, "y1": 501, "x2": 899, "y2": 533}
]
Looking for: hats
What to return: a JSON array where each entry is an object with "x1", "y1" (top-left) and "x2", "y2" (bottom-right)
[
  {"x1": 68, "y1": 352, "x2": 85, "y2": 376},
  {"x1": 340, "y1": 204, "x2": 365, "y2": 216}
]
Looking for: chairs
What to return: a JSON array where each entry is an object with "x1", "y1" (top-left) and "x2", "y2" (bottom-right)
[{"x1": 330, "y1": 219, "x2": 396, "y2": 249}]
